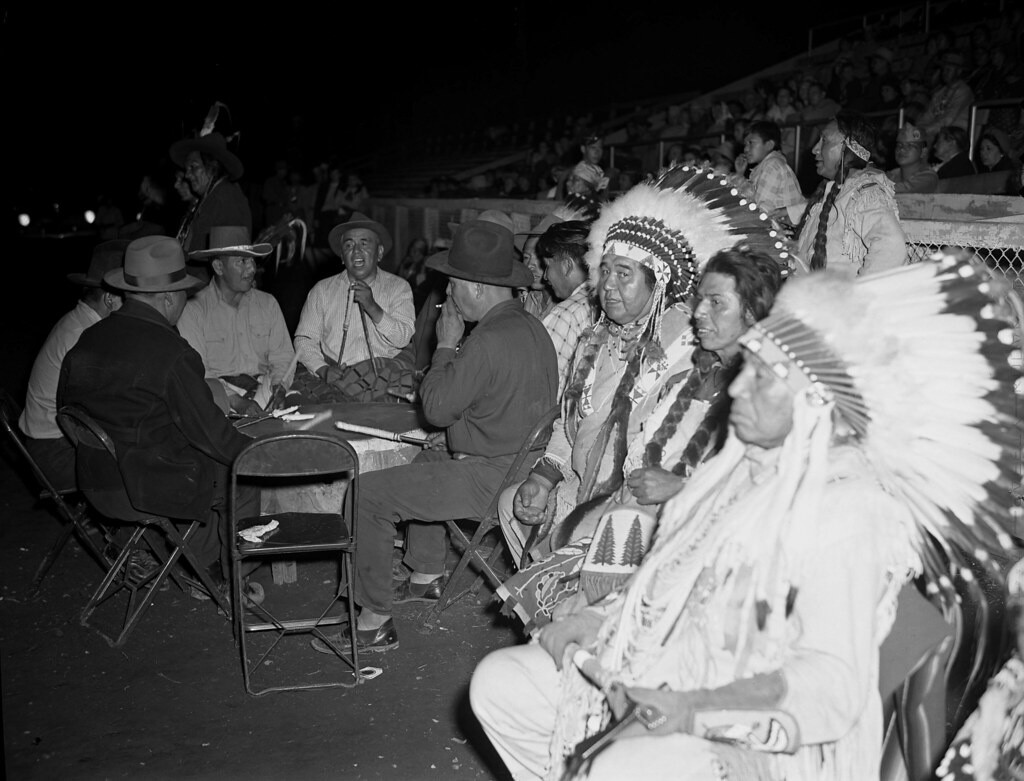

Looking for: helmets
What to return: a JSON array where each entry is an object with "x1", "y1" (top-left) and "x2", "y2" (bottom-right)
[{"x1": 752, "y1": 73, "x2": 785, "y2": 98}]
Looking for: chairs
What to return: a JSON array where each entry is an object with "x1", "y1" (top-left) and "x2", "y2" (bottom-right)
[
  {"x1": 0, "y1": 385, "x2": 128, "y2": 601},
  {"x1": 229, "y1": 430, "x2": 360, "y2": 695},
  {"x1": 422, "y1": 404, "x2": 563, "y2": 639},
  {"x1": 54, "y1": 404, "x2": 229, "y2": 651}
]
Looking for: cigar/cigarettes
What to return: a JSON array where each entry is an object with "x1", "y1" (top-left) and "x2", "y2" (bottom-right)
[{"x1": 435, "y1": 304, "x2": 445, "y2": 308}]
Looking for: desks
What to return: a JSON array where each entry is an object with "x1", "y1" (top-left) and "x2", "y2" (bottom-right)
[
  {"x1": 231, "y1": 400, "x2": 443, "y2": 633},
  {"x1": 335, "y1": 27, "x2": 1024, "y2": 202}
]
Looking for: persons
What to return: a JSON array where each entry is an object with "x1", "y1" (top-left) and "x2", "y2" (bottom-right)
[{"x1": 16, "y1": 53, "x2": 1023, "y2": 781}]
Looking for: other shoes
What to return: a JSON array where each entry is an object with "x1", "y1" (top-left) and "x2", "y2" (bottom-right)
[
  {"x1": 191, "y1": 567, "x2": 226, "y2": 600},
  {"x1": 217, "y1": 574, "x2": 264, "y2": 616},
  {"x1": 104, "y1": 529, "x2": 170, "y2": 592}
]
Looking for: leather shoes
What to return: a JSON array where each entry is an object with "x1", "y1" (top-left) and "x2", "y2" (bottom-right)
[
  {"x1": 392, "y1": 575, "x2": 445, "y2": 605},
  {"x1": 312, "y1": 615, "x2": 400, "y2": 655}
]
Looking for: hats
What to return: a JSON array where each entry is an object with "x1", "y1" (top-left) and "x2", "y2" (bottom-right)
[
  {"x1": 104, "y1": 234, "x2": 205, "y2": 293},
  {"x1": 984, "y1": 128, "x2": 1011, "y2": 156},
  {"x1": 448, "y1": 209, "x2": 524, "y2": 262},
  {"x1": 897, "y1": 122, "x2": 927, "y2": 143},
  {"x1": 935, "y1": 53, "x2": 965, "y2": 71},
  {"x1": 67, "y1": 239, "x2": 135, "y2": 288},
  {"x1": 188, "y1": 226, "x2": 273, "y2": 258},
  {"x1": 329, "y1": 211, "x2": 393, "y2": 258},
  {"x1": 171, "y1": 133, "x2": 237, "y2": 181},
  {"x1": 707, "y1": 143, "x2": 735, "y2": 165},
  {"x1": 514, "y1": 212, "x2": 564, "y2": 235},
  {"x1": 425, "y1": 219, "x2": 534, "y2": 288},
  {"x1": 870, "y1": 47, "x2": 894, "y2": 66}
]
